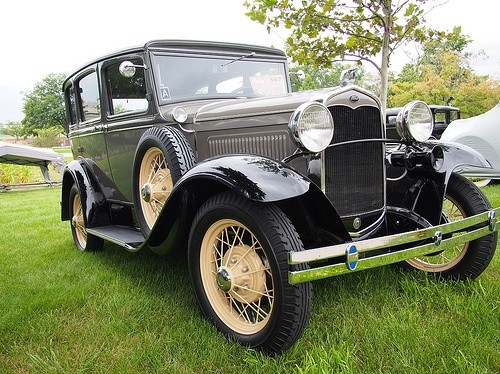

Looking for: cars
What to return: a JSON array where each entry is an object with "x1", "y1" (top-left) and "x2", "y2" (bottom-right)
[
  {"x1": 440, "y1": 100, "x2": 500, "y2": 187},
  {"x1": 386, "y1": 105, "x2": 461, "y2": 140},
  {"x1": 59, "y1": 38, "x2": 500, "y2": 358}
]
